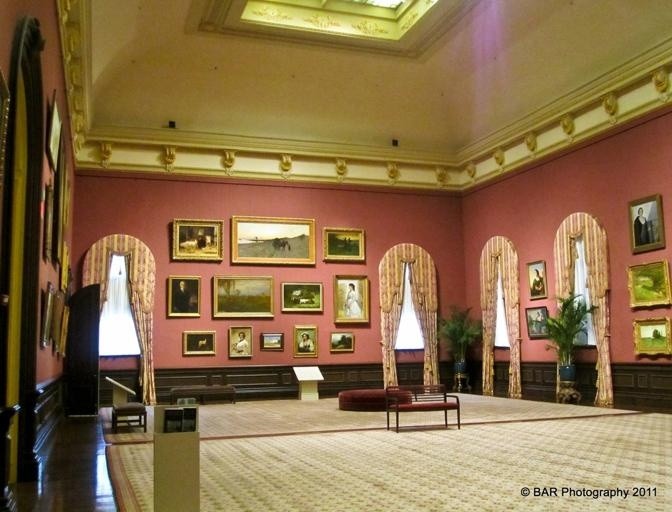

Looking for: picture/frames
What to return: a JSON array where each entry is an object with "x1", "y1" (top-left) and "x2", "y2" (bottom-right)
[
  {"x1": 526, "y1": 260, "x2": 548, "y2": 301},
  {"x1": 40, "y1": 87, "x2": 72, "y2": 356},
  {"x1": 525, "y1": 306, "x2": 551, "y2": 339},
  {"x1": 625, "y1": 259, "x2": 672, "y2": 308},
  {"x1": 632, "y1": 316, "x2": 672, "y2": 357},
  {"x1": 167, "y1": 215, "x2": 369, "y2": 358},
  {"x1": 627, "y1": 193, "x2": 666, "y2": 255}
]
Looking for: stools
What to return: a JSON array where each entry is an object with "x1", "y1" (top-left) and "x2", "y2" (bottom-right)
[
  {"x1": 339, "y1": 390, "x2": 412, "y2": 411},
  {"x1": 111, "y1": 402, "x2": 148, "y2": 432}
]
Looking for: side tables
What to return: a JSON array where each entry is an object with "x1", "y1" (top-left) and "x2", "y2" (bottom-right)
[
  {"x1": 558, "y1": 381, "x2": 582, "y2": 405},
  {"x1": 453, "y1": 374, "x2": 472, "y2": 394}
]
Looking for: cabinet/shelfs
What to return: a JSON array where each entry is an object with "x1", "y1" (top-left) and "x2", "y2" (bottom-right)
[{"x1": 154, "y1": 404, "x2": 200, "y2": 512}]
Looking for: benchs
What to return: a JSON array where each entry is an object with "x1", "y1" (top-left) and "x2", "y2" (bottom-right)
[
  {"x1": 386, "y1": 384, "x2": 461, "y2": 433},
  {"x1": 170, "y1": 385, "x2": 236, "y2": 405}
]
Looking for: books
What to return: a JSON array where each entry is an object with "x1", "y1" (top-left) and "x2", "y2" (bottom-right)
[{"x1": 164, "y1": 406, "x2": 196, "y2": 433}]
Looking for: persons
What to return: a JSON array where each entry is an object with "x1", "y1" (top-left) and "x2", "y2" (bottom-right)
[
  {"x1": 172, "y1": 281, "x2": 197, "y2": 312},
  {"x1": 343, "y1": 283, "x2": 362, "y2": 319},
  {"x1": 299, "y1": 332, "x2": 314, "y2": 352},
  {"x1": 234, "y1": 332, "x2": 249, "y2": 356},
  {"x1": 533, "y1": 268, "x2": 545, "y2": 295},
  {"x1": 634, "y1": 207, "x2": 650, "y2": 246}
]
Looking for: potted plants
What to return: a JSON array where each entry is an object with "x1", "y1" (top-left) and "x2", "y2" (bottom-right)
[
  {"x1": 435, "y1": 307, "x2": 483, "y2": 374},
  {"x1": 532, "y1": 291, "x2": 599, "y2": 381}
]
